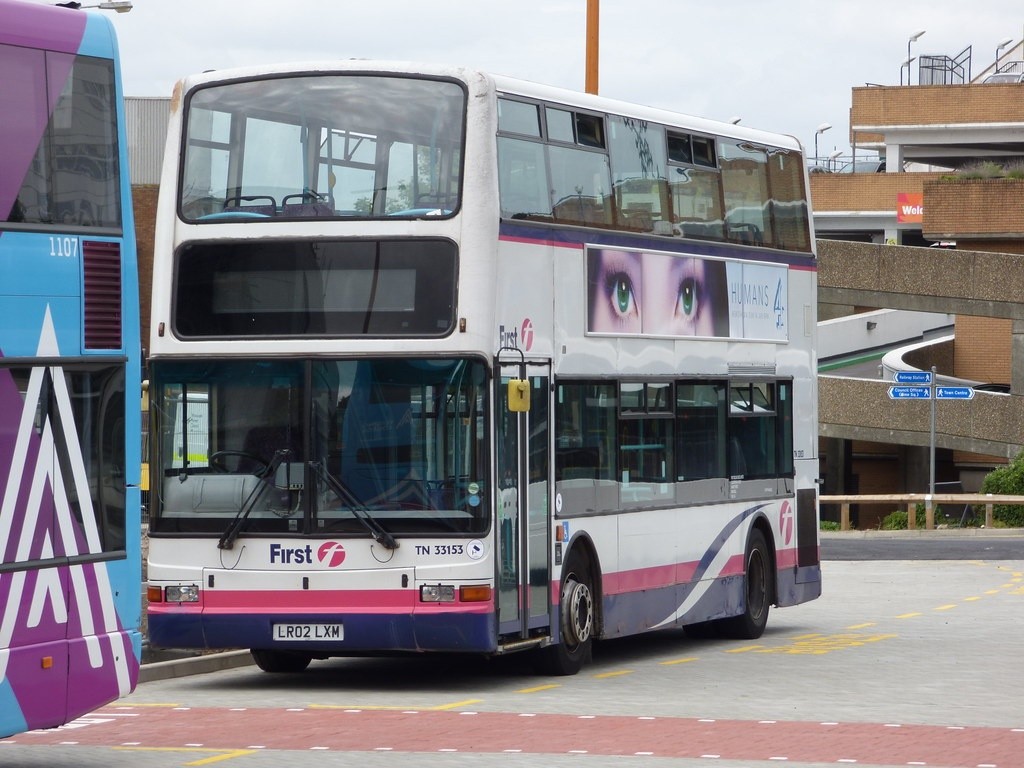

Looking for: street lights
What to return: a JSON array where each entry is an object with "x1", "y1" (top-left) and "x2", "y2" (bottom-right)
[
  {"x1": 898, "y1": 29, "x2": 928, "y2": 86},
  {"x1": 994, "y1": 39, "x2": 1012, "y2": 72},
  {"x1": 815, "y1": 128, "x2": 831, "y2": 176}
]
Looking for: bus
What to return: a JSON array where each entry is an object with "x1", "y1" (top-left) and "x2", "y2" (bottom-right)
[
  {"x1": 0, "y1": 0, "x2": 142, "y2": 738},
  {"x1": 146, "y1": 64, "x2": 823, "y2": 682}
]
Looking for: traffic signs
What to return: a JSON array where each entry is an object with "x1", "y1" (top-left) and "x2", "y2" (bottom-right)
[
  {"x1": 936, "y1": 386, "x2": 976, "y2": 399},
  {"x1": 892, "y1": 370, "x2": 932, "y2": 384},
  {"x1": 887, "y1": 385, "x2": 931, "y2": 399}
]
